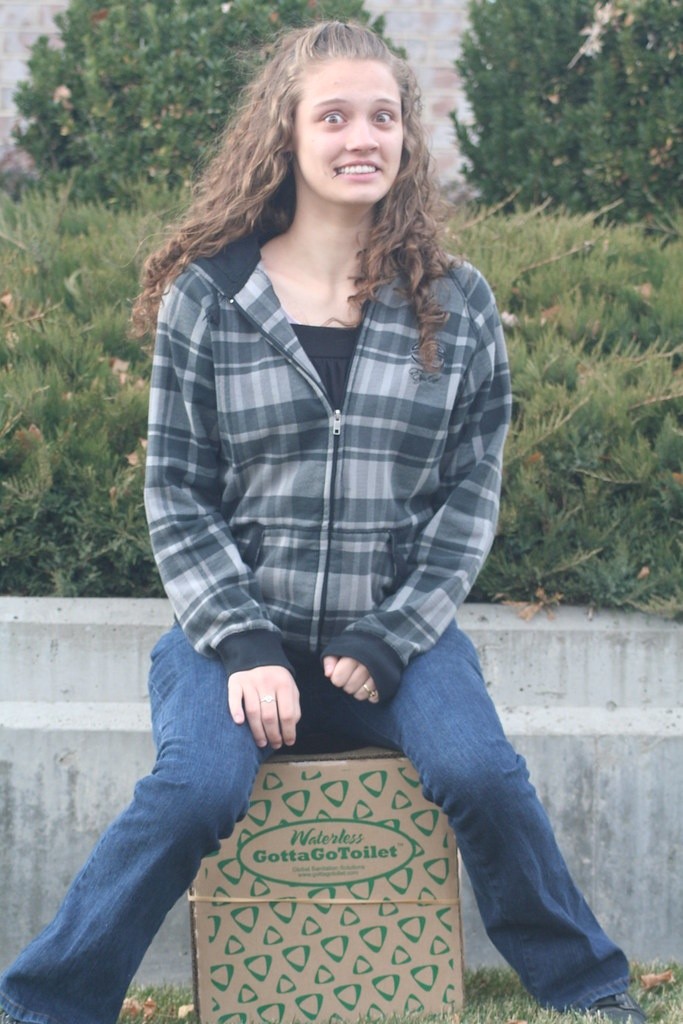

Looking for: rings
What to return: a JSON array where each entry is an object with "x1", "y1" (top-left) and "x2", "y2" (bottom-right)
[
  {"x1": 361, "y1": 684, "x2": 375, "y2": 698},
  {"x1": 258, "y1": 695, "x2": 276, "y2": 709}
]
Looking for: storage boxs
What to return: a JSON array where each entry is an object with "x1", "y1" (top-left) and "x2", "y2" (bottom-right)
[{"x1": 188, "y1": 746, "x2": 466, "y2": 1024}]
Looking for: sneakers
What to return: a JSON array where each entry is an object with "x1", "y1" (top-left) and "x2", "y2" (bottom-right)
[
  {"x1": 584, "y1": 991, "x2": 649, "y2": 1024},
  {"x1": 0, "y1": 1010, "x2": 22, "y2": 1024}
]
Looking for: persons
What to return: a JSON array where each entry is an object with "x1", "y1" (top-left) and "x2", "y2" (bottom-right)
[{"x1": 0, "y1": 20, "x2": 637, "y2": 1024}]
